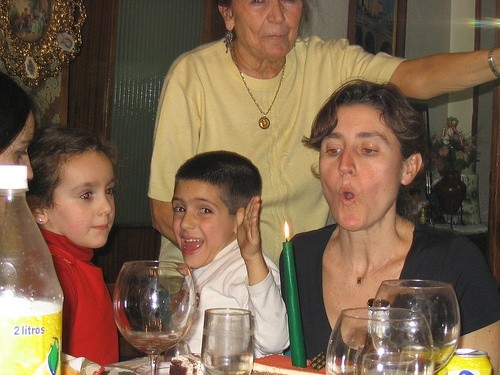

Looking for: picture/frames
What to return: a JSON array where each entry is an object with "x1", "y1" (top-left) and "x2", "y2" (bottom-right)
[{"x1": 347, "y1": 0, "x2": 407, "y2": 58}]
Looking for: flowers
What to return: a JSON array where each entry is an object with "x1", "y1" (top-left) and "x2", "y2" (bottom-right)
[{"x1": 430, "y1": 116, "x2": 481, "y2": 175}]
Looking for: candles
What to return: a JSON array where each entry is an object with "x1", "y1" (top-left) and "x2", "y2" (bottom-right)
[{"x1": 283, "y1": 238, "x2": 306, "y2": 368}]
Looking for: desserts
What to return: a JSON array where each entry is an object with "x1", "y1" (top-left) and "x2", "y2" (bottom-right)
[{"x1": 170, "y1": 353, "x2": 204, "y2": 375}]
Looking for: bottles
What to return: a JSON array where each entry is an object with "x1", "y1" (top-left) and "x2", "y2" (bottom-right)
[
  {"x1": 0, "y1": 162, "x2": 65, "y2": 374},
  {"x1": 355, "y1": 299, "x2": 402, "y2": 375}
]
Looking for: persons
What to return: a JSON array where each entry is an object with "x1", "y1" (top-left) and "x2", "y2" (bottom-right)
[
  {"x1": 279, "y1": 77, "x2": 500, "y2": 375},
  {"x1": 0, "y1": 67, "x2": 35, "y2": 254},
  {"x1": 26, "y1": 125, "x2": 120, "y2": 368},
  {"x1": 148, "y1": 0, "x2": 500, "y2": 280},
  {"x1": 165, "y1": 149, "x2": 289, "y2": 359}
]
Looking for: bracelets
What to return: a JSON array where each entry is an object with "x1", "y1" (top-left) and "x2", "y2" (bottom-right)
[{"x1": 487, "y1": 48, "x2": 500, "y2": 78}]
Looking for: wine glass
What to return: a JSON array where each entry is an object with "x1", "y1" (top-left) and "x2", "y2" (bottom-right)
[{"x1": 113, "y1": 260, "x2": 196, "y2": 375}]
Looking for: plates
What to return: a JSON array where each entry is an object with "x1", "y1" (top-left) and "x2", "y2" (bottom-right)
[
  {"x1": 101, "y1": 366, "x2": 140, "y2": 375},
  {"x1": 134, "y1": 361, "x2": 172, "y2": 375}
]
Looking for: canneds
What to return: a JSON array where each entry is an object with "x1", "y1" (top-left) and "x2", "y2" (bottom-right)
[{"x1": 437, "y1": 348, "x2": 493, "y2": 375}]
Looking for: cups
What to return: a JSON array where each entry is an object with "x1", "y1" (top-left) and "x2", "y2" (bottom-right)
[
  {"x1": 362, "y1": 352, "x2": 418, "y2": 375},
  {"x1": 201, "y1": 308, "x2": 254, "y2": 375},
  {"x1": 162, "y1": 339, "x2": 191, "y2": 363},
  {"x1": 369, "y1": 279, "x2": 461, "y2": 375},
  {"x1": 328, "y1": 308, "x2": 434, "y2": 375}
]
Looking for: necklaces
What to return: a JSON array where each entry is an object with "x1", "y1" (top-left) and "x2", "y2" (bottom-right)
[
  {"x1": 232, "y1": 45, "x2": 287, "y2": 129},
  {"x1": 335, "y1": 233, "x2": 404, "y2": 284}
]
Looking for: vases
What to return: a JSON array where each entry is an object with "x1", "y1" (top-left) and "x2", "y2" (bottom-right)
[{"x1": 431, "y1": 170, "x2": 482, "y2": 225}]
